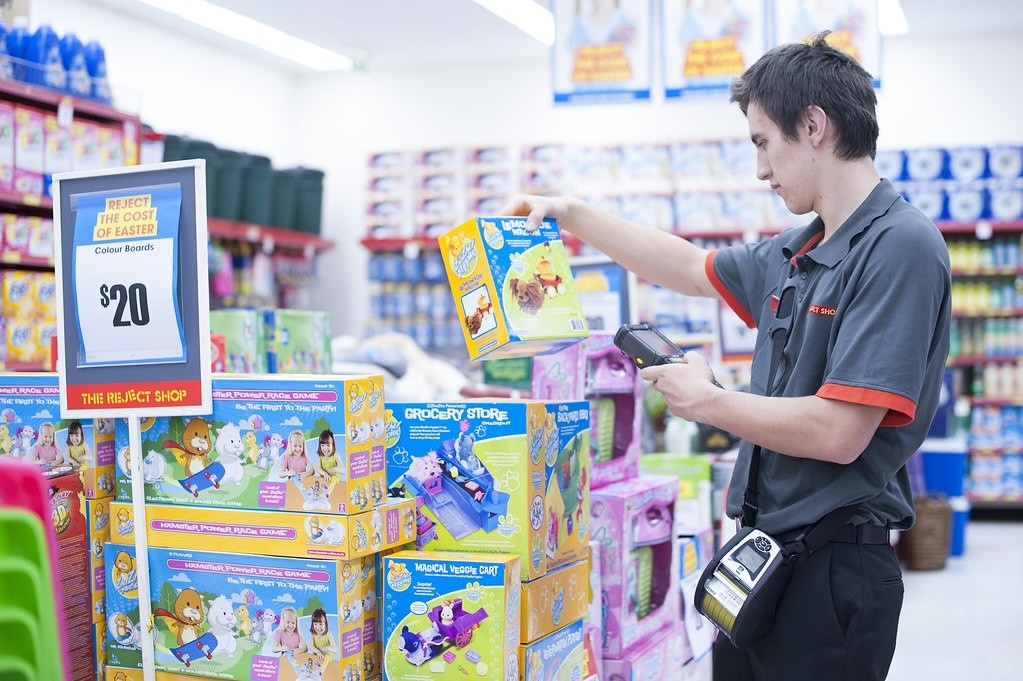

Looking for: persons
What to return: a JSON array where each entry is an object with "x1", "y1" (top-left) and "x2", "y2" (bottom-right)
[
  {"x1": 501, "y1": 29, "x2": 951, "y2": 681},
  {"x1": 316, "y1": 430, "x2": 343, "y2": 482},
  {"x1": 32, "y1": 423, "x2": 63, "y2": 466},
  {"x1": 272, "y1": 607, "x2": 308, "y2": 656},
  {"x1": 66, "y1": 422, "x2": 92, "y2": 466},
  {"x1": 279, "y1": 431, "x2": 315, "y2": 481},
  {"x1": 309, "y1": 608, "x2": 337, "y2": 659}
]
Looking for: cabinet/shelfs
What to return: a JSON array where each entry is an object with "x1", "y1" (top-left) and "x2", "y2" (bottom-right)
[
  {"x1": 362, "y1": 140, "x2": 806, "y2": 352},
  {"x1": 912, "y1": 221, "x2": 1022, "y2": 509},
  {"x1": 0, "y1": 79, "x2": 138, "y2": 370}
]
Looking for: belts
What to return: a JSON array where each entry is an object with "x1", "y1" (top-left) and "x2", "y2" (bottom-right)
[{"x1": 779, "y1": 521, "x2": 888, "y2": 543}]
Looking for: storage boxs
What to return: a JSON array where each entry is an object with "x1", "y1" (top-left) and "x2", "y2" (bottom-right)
[
  {"x1": 904, "y1": 373, "x2": 970, "y2": 568},
  {"x1": 1, "y1": 214, "x2": 732, "y2": 681}
]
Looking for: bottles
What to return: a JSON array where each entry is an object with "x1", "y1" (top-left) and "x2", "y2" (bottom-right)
[
  {"x1": 0, "y1": 17, "x2": 111, "y2": 105},
  {"x1": 945, "y1": 233, "x2": 1023, "y2": 267},
  {"x1": 951, "y1": 276, "x2": 1023, "y2": 311},
  {"x1": 943, "y1": 362, "x2": 1023, "y2": 398},
  {"x1": 949, "y1": 317, "x2": 1023, "y2": 355}
]
